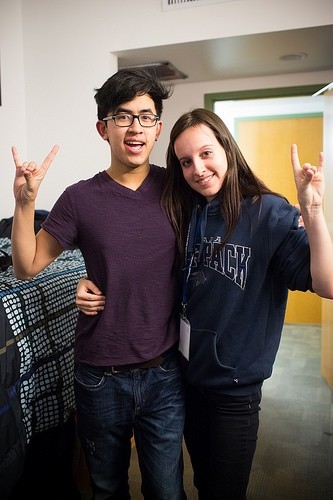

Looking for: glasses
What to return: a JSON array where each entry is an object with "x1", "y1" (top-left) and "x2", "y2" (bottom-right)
[{"x1": 102, "y1": 113, "x2": 160, "y2": 127}]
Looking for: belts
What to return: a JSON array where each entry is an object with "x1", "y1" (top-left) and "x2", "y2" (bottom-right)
[{"x1": 97, "y1": 356, "x2": 174, "y2": 375}]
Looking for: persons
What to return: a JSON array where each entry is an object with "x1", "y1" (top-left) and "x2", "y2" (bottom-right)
[
  {"x1": 9, "y1": 67, "x2": 202, "y2": 500},
  {"x1": 74, "y1": 107, "x2": 333, "y2": 500}
]
